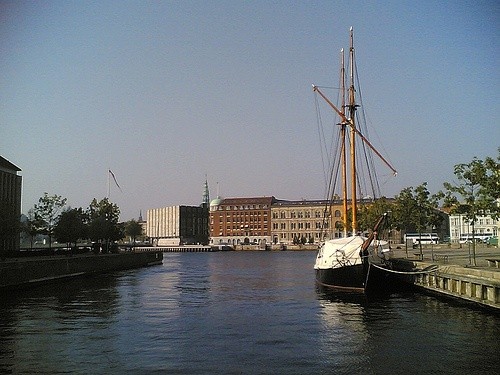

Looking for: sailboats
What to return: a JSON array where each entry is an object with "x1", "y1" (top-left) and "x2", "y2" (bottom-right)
[{"x1": 309, "y1": 26, "x2": 403, "y2": 297}]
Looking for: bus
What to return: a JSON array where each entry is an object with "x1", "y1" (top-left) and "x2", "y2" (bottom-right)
[
  {"x1": 459, "y1": 233, "x2": 493, "y2": 244},
  {"x1": 404, "y1": 233, "x2": 440, "y2": 245}
]
